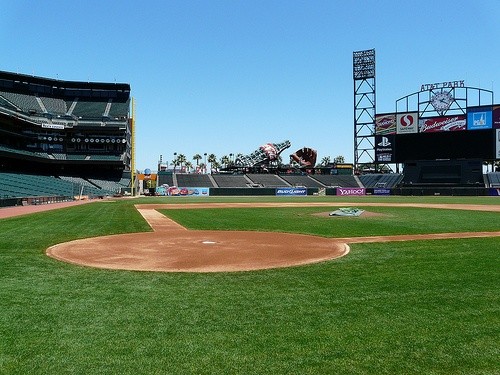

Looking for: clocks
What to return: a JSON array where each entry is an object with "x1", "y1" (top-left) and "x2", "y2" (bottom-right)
[{"x1": 434, "y1": 94, "x2": 450, "y2": 108}]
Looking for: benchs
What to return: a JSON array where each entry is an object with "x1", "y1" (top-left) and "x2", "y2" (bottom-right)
[{"x1": 0, "y1": 173, "x2": 110, "y2": 207}]
